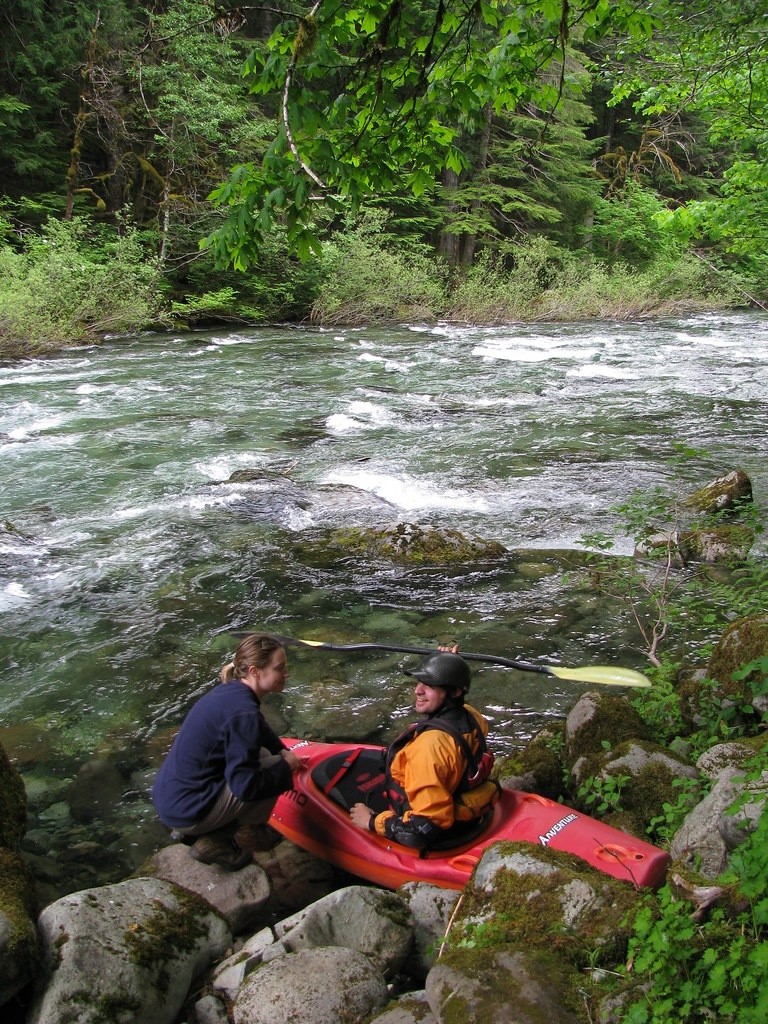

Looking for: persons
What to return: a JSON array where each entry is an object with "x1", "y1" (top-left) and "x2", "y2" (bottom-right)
[
  {"x1": 351, "y1": 644, "x2": 501, "y2": 851},
  {"x1": 152, "y1": 634, "x2": 310, "y2": 872}
]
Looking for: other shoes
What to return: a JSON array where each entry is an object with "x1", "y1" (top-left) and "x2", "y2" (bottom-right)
[
  {"x1": 188, "y1": 838, "x2": 255, "y2": 871},
  {"x1": 168, "y1": 830, "x2": 191, "y2": 847}
]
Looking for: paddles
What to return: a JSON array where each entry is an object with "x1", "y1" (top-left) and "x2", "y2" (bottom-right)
[{"x1": 224, "y1": 630, "x2": 654, "y2": 687}]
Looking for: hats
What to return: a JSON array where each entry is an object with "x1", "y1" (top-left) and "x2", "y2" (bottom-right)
[{"x1": 401, "y1": 651, "x2": 473, "y2": 695}]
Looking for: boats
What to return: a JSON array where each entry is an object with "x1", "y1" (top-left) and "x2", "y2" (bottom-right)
[{"x1": 265, "y1": 739, "x2": 670, "y2": 901}]
